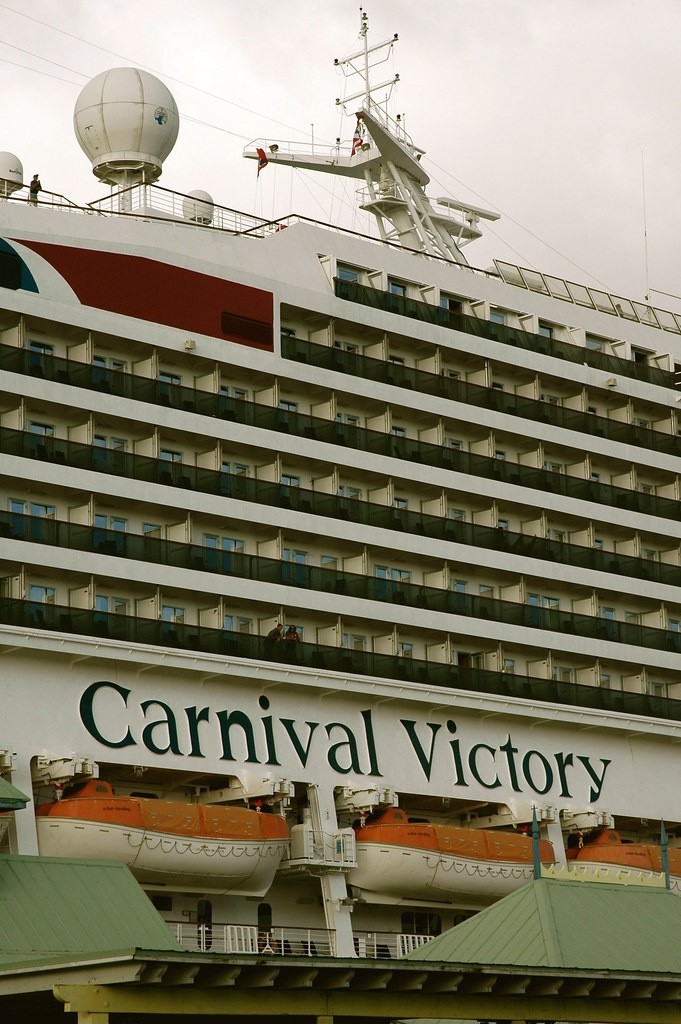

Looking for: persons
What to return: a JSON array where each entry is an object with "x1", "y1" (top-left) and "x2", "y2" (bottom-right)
[
  {"x1": 284, "y1": 625, "x2": 300, "y2": 664},
  {"x1": 264, "y1": 624, "x2": 283, "y2": 659},
  {"x1": 27, "y1": 174, "x2": 42, "y2": 207}
]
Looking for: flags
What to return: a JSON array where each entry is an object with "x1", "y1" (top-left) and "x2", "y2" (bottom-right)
[
  {"x1": 256, "y1": 148, "x2": 268, "y2": 179},
  {"x1": 349, "y1": 115, "x2": 366, "y2": 157}
]
